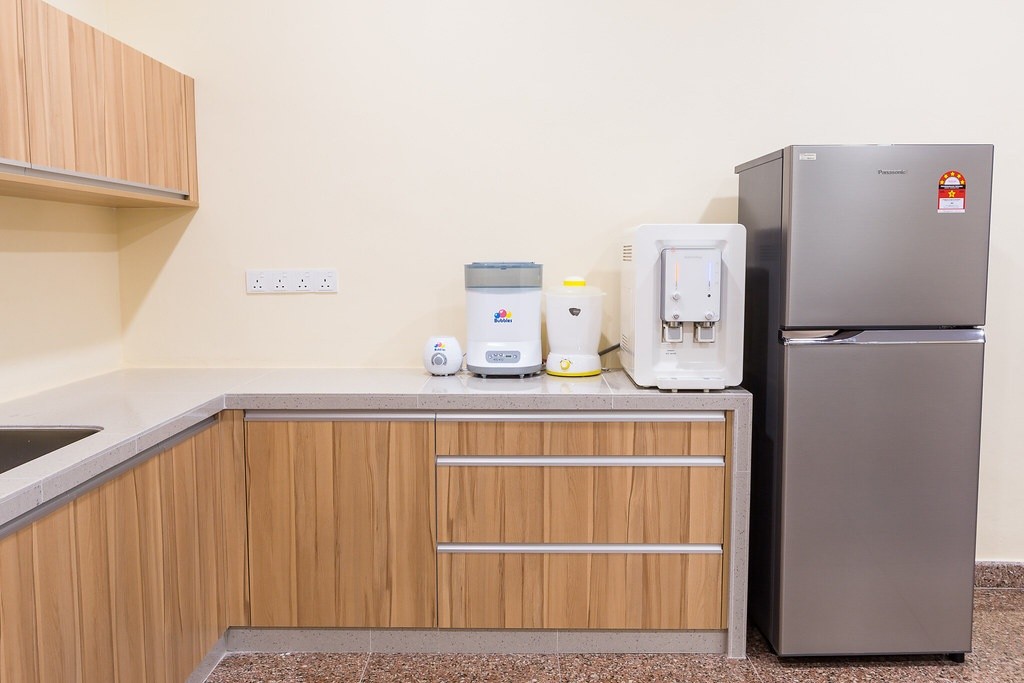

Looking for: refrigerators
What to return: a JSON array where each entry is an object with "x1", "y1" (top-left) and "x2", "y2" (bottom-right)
[{"x1": 734, "y1": 143, "x2": 996, "y2": 658}]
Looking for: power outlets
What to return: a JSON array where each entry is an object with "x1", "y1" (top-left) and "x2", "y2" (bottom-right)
[{"x1": 245, "y1": 268, "x2": 338, "y2": 294}]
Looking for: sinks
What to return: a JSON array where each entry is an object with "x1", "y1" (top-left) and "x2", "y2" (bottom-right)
[{"x1": 0, "y1": 424, "x2": 107, "y2": 476}]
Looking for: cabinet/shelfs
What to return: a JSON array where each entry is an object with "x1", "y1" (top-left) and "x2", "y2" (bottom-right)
[
  {"x1": 0, "y1": 0, "x2": 199, "y2": 208},
  {"x1": 223, "y1": 409, "x2": 733, "y2": 631},
  {"x1": 0, "y1": 413, "x2": 227, "y2": 683}
]
